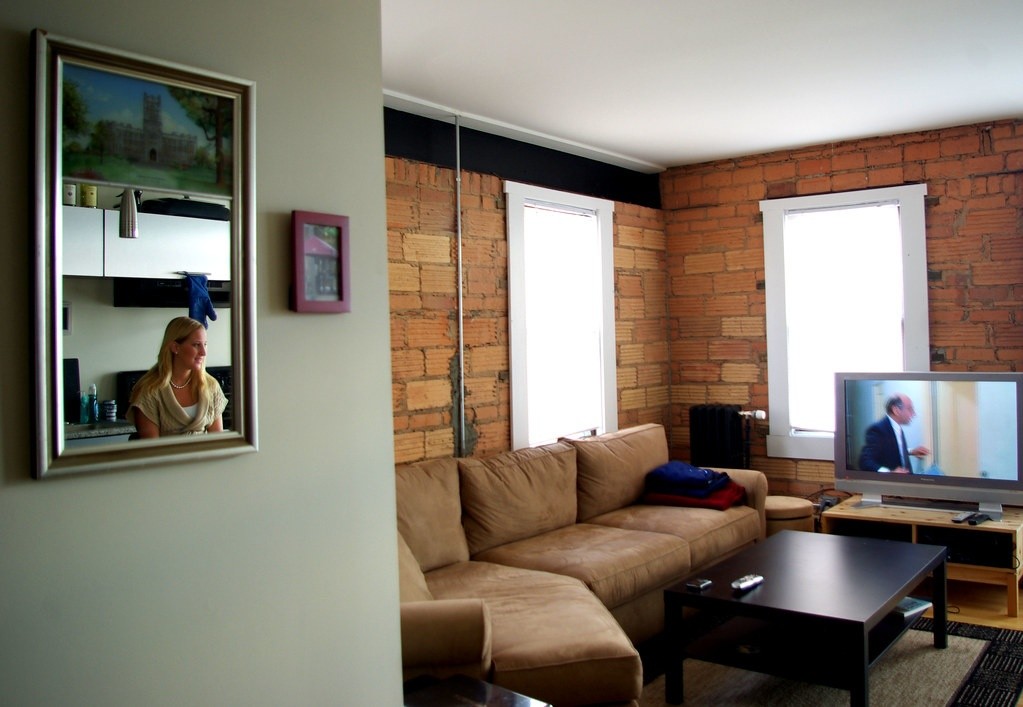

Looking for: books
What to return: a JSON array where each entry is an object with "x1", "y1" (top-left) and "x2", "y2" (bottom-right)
[{"x1": 891, "y1": 596, "x2": 932, "y2": 617}]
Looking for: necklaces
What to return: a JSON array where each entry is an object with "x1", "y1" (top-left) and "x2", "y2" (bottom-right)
[{"x1": 170, "y1": 377, "x2": 191, "y2": 389}]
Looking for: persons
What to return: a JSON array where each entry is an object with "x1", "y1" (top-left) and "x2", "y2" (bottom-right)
[
  {"x1": 858, "y1": 394, "x2": 930, "y2": 474},
  {"x1": 127, "y1": 317, "x2": 228, "y2": 439}
]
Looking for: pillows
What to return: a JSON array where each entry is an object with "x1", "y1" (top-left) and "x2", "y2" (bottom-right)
[
  {"x1": 455, "y1": 440, "x2": 577, "y2": 555},
  {"x1": 557, "y1": 422, "x2": 668, "y2": 524},
  {"x1": 396, "y1": 531, "x2": 435, "y2": 602},
  {"x1": 392, "y1": 456, "x2": 469, "y2": 573}
]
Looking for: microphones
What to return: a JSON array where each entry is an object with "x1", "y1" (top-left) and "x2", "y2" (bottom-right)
[{"x1": 739, "y1": 410, "x2": 766, "y2": 420}]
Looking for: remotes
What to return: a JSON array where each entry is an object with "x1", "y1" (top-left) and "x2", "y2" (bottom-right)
[
  {"x1": 951, "y1": 512, "x2": 976, "y2": 523},
  {"x1": 968, "y1": 514, "x2": 989, "y2": 525},
  {"x1": 732, "y1": 576, "x2": 765, "y2": 589}
]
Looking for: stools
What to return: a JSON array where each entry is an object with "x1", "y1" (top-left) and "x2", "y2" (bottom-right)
[{"x1": 765, "y1": 495, "x2": 814, "y2": 533}]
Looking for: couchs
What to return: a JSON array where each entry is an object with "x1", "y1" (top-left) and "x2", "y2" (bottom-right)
[{"x1": 395, "y1": 420, "x2": 768, "y2": 707}]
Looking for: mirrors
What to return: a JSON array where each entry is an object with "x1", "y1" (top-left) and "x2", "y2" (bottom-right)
[{"x1": 29, "y1": 27, "x2": 259, "y2": 481}]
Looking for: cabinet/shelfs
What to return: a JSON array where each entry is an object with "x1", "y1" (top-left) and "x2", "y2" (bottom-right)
[{"x1": 821, "y1": 494, "x2": 1023, "y2": 618}]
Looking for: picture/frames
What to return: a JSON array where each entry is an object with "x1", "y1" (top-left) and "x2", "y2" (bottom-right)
[{"x1": 289, "y1": 209, "x2": 351, "y2": 314}]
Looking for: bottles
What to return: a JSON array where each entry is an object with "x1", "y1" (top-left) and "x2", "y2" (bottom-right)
[{"x1": 88, "y1": 384, "x2": 99, "y2": 423}]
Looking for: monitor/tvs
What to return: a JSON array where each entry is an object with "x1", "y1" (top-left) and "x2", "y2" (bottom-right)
[{"x1": 833, "y1": 372, "x2": 1023, "y2": 518}]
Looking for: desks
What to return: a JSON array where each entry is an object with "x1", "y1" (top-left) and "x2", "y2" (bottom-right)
[{"x1": 664, "y1": 530, "x2": 948, "y2": 707}]
